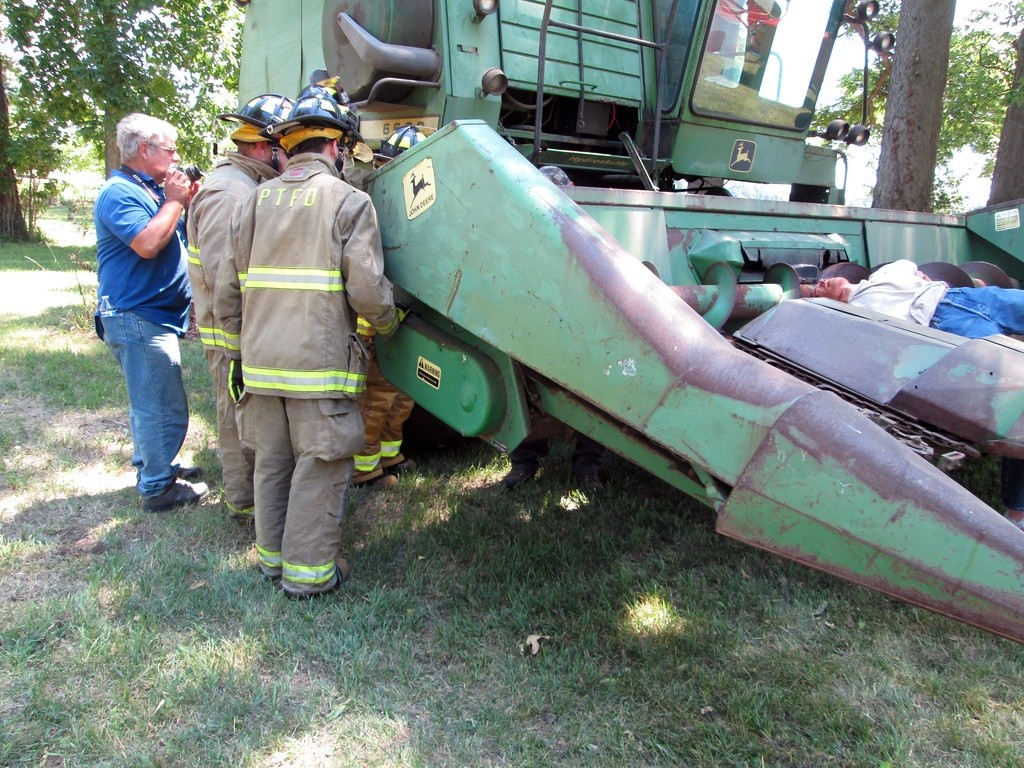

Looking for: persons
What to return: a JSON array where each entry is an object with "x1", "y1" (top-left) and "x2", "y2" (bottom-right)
[
  {"x1": 96, "y1": 113, "x2": 211, "y2": 511},
  {"x1": 503, "y1": 166, "x2": 605, "y2": 493},
  {"x1": 214, "y1": 79, "x2": 400, "y2": 601},
  {"x1": 347, "y1": 127, "x2": 428, "y2": 491},
  {"x1": 186, "y1": 95, "x2": 293, "y2": 520},
  {"x1": 810, "y1": 259, "x2": 1024, "y2": 527}
]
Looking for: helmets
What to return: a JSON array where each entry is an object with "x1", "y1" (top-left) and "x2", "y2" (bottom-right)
[
  {"x1": 371, "y1": 124, "x2": 427, "y2": 171},
  {"x1": 310, "y1": 69, "x2": 342, "y2": 88},
  {"x1": 297, "y1": 85, "x2": 350, "y2": 115},
  {"x1": 216, "y1": 93, "x2": 295, "y2": 143},
  {"x1": 257, "y1": 95, "x2": 351, "y2": 153},
  {"x1": 338, "y1": 105, "x2": 365, "y2": 151}
]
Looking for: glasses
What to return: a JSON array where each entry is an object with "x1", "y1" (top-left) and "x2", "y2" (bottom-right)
[{"x1": 146, "y1": 143, "x2": 177, "y2": 155}]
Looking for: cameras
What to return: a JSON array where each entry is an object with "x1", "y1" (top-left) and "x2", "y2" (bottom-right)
[{"x1": 170, "y1": 163, "x2": 204, "y2": 182}]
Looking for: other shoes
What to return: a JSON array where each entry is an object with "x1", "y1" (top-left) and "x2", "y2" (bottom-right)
[
  {"x1": 142, "y1": 477, "x2": 211, "y2": 512},
  {"x1": 174, "y1": 461, "x2": 203, "y2": 481},
  {"x1": 244, "y1": 548, "x2": 283, "y2": 582},
  {"x1": 282, "y1": 558, "x2": 351, "y2": 600}
]
